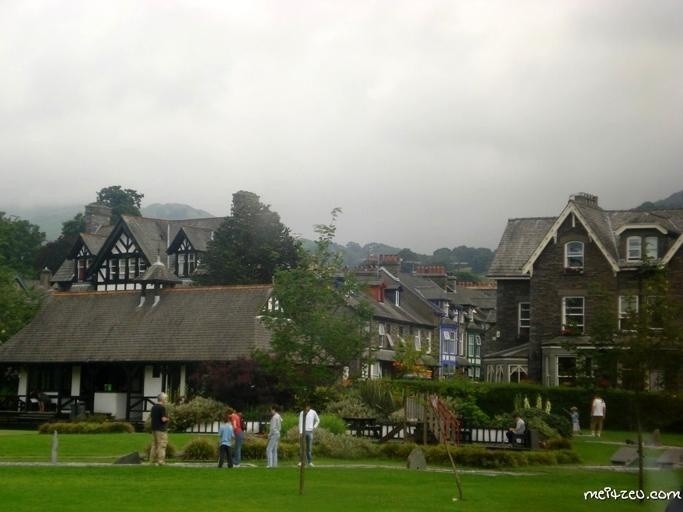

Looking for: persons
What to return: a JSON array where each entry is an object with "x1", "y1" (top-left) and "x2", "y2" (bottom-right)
[
  {"x1": 266, "y1": 404, "x2": 282, "y2": 468},
  {"x1": 298, "y1": 402, "x2": 320, "y2": 467},
  {"x1": 570, "y1": 407, "x2": 580, "y2": 432},
  {"x1": 149, "y1": 392, "x2": 170, "y2": 465},
  {"x1": 590, "y1": 393, "x2": 606, "y2": 437},
  {"x1": 218, "y1": 408, "x2": 242, "y2": 468},
  {"x1": 506, "y1": 414, "x2": 525, "y2": 447}
]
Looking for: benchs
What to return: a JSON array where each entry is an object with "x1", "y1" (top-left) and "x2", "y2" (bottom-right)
[
  {"x1": 343, "y1": 416, "x2": 383, "y2": 439},
  {"x1": 512, "y1": 430, "x2": 531, "y2": 448}
]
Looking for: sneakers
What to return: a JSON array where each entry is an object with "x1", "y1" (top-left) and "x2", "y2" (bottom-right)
[{"x1": 297, "y1": 462, "x2": 314, "y2": 467}]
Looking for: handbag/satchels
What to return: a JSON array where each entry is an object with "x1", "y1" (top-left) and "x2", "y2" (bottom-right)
[{"x1": 240, "y1": 417, "x2": 246, "y2": 430}]
